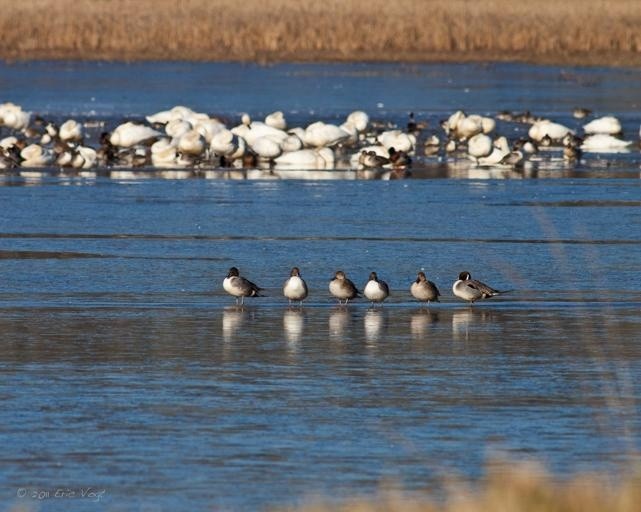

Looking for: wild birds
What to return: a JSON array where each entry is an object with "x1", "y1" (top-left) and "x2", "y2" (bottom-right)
[
  {"x1": 363, "y1": 272, "x2": 390, "y2": 308},
  {"x1": 410, "y1": 272, "x2": 442, "y2": 306},
  {"x1": 453, "y1": 267, "x2": 516, "y2": 308},
  {"x1": 224, "y1": 267, "x2": 269, "y2": 305},
  {"x1": 329, "y1": 271, "x2": 363, "y2": 306},
  {"x1": 0, "y1": 103, "x2": 641, "y2": 169},
  {"x1": 283, "y1": 267, "x2": 309, "y2": 307}
]
